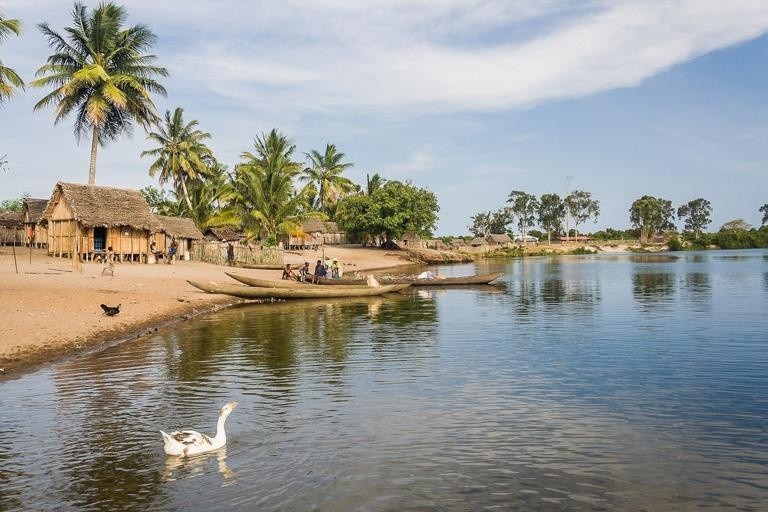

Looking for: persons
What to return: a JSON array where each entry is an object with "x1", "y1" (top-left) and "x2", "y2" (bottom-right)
[
  {"x1": 227, "y1": 242, "x2": 234, "y2": 267},
  {"x1": 102, "y1": 247, "x2": 114, "y2": 276},
  {"x1": 150, "y1": 241, "x2": 162, "y2": 264},
  {"x1": 282, "y1": 256, "x2": 340, "y2": 284},
  {"x1": 167, "y1": 238, "x2": 177, "y2": 264}
]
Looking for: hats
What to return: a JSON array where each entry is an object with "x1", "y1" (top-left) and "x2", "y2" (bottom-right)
[{"x1": 332, "y1": 258, "x2": 339, "y2": 262}]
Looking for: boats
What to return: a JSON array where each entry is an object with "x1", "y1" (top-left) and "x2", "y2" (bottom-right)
[
  {"x1": 185, "y1": 269, "x2": 411, "y2": 298},
  {"x1": 366, "y1": 270, "x2": 504, "y2": 288}
]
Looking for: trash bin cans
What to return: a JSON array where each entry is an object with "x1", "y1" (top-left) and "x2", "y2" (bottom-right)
[
  {"x1": 279, "y1": 242, "x2": 283, "y2": 248},
  {"x1": 184, "y1": 251, "x2": 190, "y2": 261}
]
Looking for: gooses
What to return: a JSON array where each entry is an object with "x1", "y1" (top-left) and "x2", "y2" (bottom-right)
[{"x1": 159, "y1": 401, "x2": 240, "y2": 456}]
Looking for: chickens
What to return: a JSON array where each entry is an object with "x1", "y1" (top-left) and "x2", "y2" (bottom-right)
[{"x1": 100, "y1": 303, "x2": 121, "y2": 317}]
